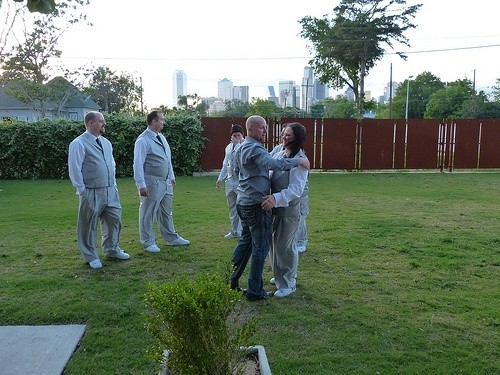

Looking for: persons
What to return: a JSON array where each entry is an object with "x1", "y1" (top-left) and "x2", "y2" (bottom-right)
[
  {"x1": 226, "y1": 115, "x2": 310, "y2": 301},
  {"x1": 260, "y1": 122, "x2": 309, "y2": 298},
  {"x1": 133, "y1": 111, "x2": 190, "y2": 252},
  {"x1": 68, "y1": 111, "x2": 130, "y2": 269},
  {"x1": 216, "y1": 125, "x2": 245, "y2": 238}
]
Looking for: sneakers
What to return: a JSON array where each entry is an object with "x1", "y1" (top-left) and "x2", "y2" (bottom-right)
[
  {"x1": 144, "y1": 244, "x2": 160, "y2": 252},
  {"x1": 167, "y1": 236, "x2": 190, "y2": 245}
]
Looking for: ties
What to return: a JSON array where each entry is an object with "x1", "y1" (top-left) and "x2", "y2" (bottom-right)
[
  {"x1": 96, "y1": 138, "x2": 102, "y2": 148},
  {"x1": 156, "y1": 135, "x2": 163, "y2": 144},
  {"x1": 232, "y1": 143, "x2": 235, "y2": 151}
]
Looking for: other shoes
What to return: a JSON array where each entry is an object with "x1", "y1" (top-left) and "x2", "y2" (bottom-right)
[
  {"x1": 269, "y1": 277, "x2": 275, "y2": 284},
  {"x1": 225, "y1": 233, "x2": 239, "y2": 238},
  {"x1": 226, "y1": 278, "x2": 248, "y2": 295},
  {"x1": 274, "y1": 286, "x2": 296, "y2": 297},
  {"x1": 104, "y1": 250, "x2": 130, "y2": 259},
  {"x1": 263, "y1": 290, "x2": 273, "y2": 298},
  {"x1": 298, "y1": 243, "x2": 306, "y2": 254},
  {"x1": 89, "y1": 258, "x2": 102, "y2": 268}
]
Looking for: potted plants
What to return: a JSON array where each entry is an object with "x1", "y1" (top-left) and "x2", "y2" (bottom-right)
[{"x1": 139, "y1": 259, "x2": 271, "y2": 375}]
